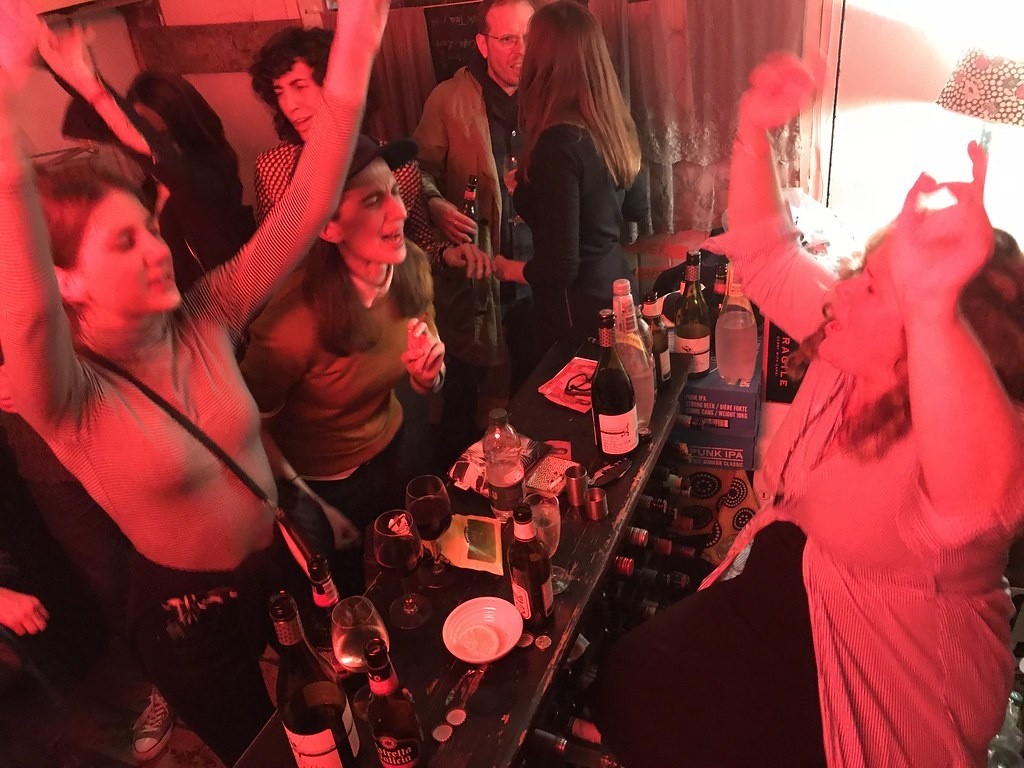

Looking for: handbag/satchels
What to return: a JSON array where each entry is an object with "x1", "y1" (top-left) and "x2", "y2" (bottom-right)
[
  {"x1": 447, "y1": 430, "x2": 552, "y2": 501},
  {"x1": 273, "y1": 478, "x2": 335, "y2": 584}
]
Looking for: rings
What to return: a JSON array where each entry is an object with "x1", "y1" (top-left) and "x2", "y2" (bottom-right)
[{"x1": 453, "y1": 221, "x2": 458, "y2": 227}]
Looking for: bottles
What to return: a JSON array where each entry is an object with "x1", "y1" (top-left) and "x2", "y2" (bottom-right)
[
  {"x1": 363, "y1": 637, "x2": 426, "y2": 768},
  {"x1": 611, "y1": 278, "x2": 654, "y2": 429},
  {"x1": 642, "y1": 292, "x2": 672, "y2": 388},
  {"x1": 456, "y1": 175, "x2": 479, "y2": 245},
  {"x1": 304, "y1": 554, "x2": 368, "y2": 694},
  {"x1": 987, "y1": 691, "x2": 1024, "y2": 768},
  {"x1": 590, "y1": 308, "x2": 640, "y2": 459},
  {"x1": 674, "y1": 249, "x2": 758, "y2": 385},
  {"x1": 481, "y1": 409, "x2": 705, "y2": 768},
  {"x1": 267, "y1": 589, "x2": 364, "y2": 768},
  {"x1": 634, "y1": 303, "x2": 657, "y2": 403}
]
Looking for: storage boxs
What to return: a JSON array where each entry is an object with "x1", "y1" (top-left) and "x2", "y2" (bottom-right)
[{"x1": 664, "y1": 339, "x2": 763, "y2": 471}]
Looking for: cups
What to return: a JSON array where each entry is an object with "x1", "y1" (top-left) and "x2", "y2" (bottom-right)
[
  {"x1": 583, "y1": 488, "x2": 608, "y2": 520},
  {"x1": 564, "y1": 465, "x2": 588, "y2": 507}
]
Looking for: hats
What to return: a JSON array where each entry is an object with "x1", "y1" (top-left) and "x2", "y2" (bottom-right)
[{"x1": 291, "y1": 133, "x2": 418, "y2": 182}]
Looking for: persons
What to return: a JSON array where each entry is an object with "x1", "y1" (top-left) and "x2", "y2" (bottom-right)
[
  {"x1": 251, "y1": 24, "x2": 491, "y2": 279},
  {"x1": 35, "y1": 15, "x2": 256, "y2": 292},
  {"x1": 588, "y1": 41, "x2": 1024, "y2": 768},
  {"x1": 492, "y1": 0, "x2": 641, "y2": 396},
  {"x1": 412, "y1": 0, "x2": 535, "y2": 422},
  {"x1": 0, "y1": 0, "x2": 390, "y2": 768},
  {"x1": 240, "y1": 133, "x2": 446, "y2": 581},
  {"x1": 0, "y1": 408, "x2": 176, "y2": 761}
]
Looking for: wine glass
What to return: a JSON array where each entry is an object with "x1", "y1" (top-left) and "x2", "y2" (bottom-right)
[
  {"x1": 502, "y1": 152, "x2": 526, "y2": 224},
  {"x1": 372, "y1": 508, "x2": 434, "y2": 630},
  {"x1": 404, "y1": 474, "x2": 459, "y2": 589},
  {"x1": 331, "y1": 595, "x2": 390, "y2": 721},
  {"x1": 523, "y1": 492, "x2": 570, "y2": 595}
]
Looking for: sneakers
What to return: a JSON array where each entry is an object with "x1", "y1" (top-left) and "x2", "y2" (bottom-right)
[{"x1": 130, "y1": 686, "x2": 174, "y2": 761}]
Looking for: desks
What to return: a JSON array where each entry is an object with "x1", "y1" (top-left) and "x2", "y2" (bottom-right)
[{"x1": 235, "y1": 254, "x2": 709, "y2": 766}]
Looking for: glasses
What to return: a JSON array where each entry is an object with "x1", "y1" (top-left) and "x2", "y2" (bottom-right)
[
  {"x1": 478, "y1": 32, "x2": 528, "y2": 50},
  {"x1": 565, "y1": 373, "x2": 592, "y2": 396}
]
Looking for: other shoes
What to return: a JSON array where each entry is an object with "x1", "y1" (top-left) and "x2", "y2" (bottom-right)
[{"x1": 441, "y1": 415, "x2": 485, "y2": 447}]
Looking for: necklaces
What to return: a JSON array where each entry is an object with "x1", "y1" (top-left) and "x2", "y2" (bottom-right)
[
  {"x1": 773, "y1": 376, "x2": 856, "y2": 508},
  {"x1": 348, "y1": 262, "x2": 391, "y2": 288}
]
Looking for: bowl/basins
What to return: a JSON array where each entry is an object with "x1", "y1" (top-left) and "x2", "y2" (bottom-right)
[{"x1": 441, "y1": 596, "x2": 524, "y2": 664}]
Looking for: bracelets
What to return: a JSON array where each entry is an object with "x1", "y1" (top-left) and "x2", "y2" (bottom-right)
[{"x1": 88, "y1": 89, "x2": 104, "y2": 103}]
